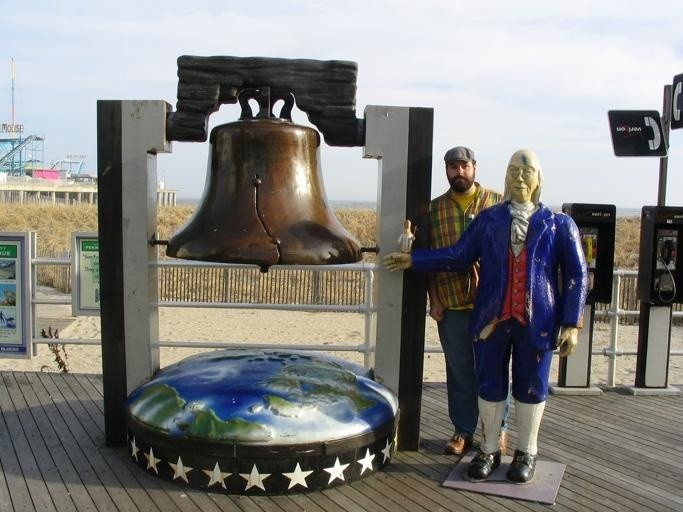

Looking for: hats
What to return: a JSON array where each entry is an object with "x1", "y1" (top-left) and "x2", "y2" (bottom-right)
[{"x1": 443, "y1": 146, "x2": 474, "y2": 162}]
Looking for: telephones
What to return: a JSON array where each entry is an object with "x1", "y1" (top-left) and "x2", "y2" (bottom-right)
[
  {"x1": 585, "y1": 235, "x2": 593, "y2": 265},
  {"x1": 664, "y1": 240, "x2": 673, "y2": 266}
]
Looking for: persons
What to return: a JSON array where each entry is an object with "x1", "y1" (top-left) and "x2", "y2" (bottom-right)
[
  {"x1": 382, "y1": 150, "x2": 588, "y2": 479},
  {"x1": 396, "y1": 219, "x2": 419, "y2": 253},
  {"x1": 429, "y1": 146, "x2": 509, "y2": 458}
]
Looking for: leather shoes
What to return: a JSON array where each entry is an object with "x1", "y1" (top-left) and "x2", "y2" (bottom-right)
[
  {"x1": 498, "y1": 430, "x2": 507, "y2": 455},
  {"x1": 445, "y1": 432, "x2": 473, "y2": 453}
]
